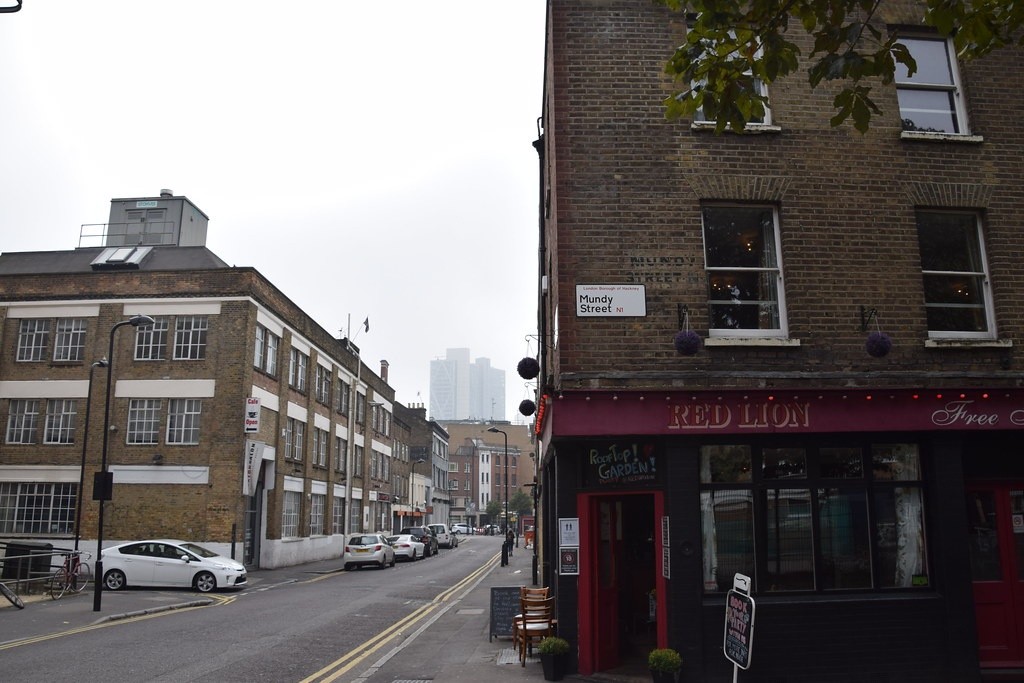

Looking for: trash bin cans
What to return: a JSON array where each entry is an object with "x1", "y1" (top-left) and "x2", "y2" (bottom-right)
[
  {"x1": 2, "y1": 541, "x2": 53, "y2": 579},
  {"x1": 526, "y1": 530, "x2": 534, "y2": 546}
]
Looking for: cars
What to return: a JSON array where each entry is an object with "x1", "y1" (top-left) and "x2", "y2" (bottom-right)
[
  {"x1": 451, "y1": 522, "x2": 503, "y2": 536},
  {"x1": 93, "y1": 538, "x2": 247, "y2": 594},
  {"x1": 385, "y1": 533, "x2": 425, "y2": 561}
]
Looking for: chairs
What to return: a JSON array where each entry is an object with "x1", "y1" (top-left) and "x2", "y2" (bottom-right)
[{"x1": 513, "y1": 585, "x2": 558, "y2": 668}]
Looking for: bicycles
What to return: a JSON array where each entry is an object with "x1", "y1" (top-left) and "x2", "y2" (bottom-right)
[{"x1": 50, "y1": 551, "x2": 93, "y2": 600}]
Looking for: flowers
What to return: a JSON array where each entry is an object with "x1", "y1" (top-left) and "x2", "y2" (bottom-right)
[
  {"x1": 519, "y1": 399, "x2": 538, "y2": 416},
  {"x1": 517, "y1": 357, "x2": 540, "y2": 380},
  {"x1": 673, "y1": 327, "x2": 701, "y2": 355},
  {"x1": 866, "y1": 331, "x2": 893, "y2": 356}
]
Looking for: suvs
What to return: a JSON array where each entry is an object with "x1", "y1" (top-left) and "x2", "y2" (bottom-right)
[
  {"x1": 426, "y1": 523, "x2": 459, "y2": 549},
  {"x1": 400, "y1": 525, "x2": 439, "y2": 556},
  {"x1": 343, "y1": 530, "x2": 396, "y2": 571}
]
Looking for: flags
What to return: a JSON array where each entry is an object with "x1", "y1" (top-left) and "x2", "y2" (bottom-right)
[{"x1": 364, "y1": 318, "x2": 369, "y2": 333}]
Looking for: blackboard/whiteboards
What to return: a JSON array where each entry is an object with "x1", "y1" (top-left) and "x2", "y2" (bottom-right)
[
  {"x1": 723, "y1": 589, "x2": 755, "y2": 670},
  {"x1": 490, "y1": 586, "x2": 527, "y2": 636}
]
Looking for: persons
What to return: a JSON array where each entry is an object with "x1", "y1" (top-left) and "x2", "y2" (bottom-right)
[
  {"x1": 507, "y1": 529, "x2": 515, "y2": 556},
  {"x1": 490, "y1": 524, "x2": 494, "y2": 535}
]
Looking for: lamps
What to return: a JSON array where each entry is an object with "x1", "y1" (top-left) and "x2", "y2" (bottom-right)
[
  {"x1": 152, "y1": 455, "x2": 163, "y2": 461},
  {"x1": 375, "y1": 484, "x2": 381, "y2": 489},
  {"x1": 341, "y1": 476, "x2": 347, "y2": 481},
  {"x1": 529, "y1": 451, "x2": 537, "y2": 458},
  {"x1": 290, "y1": 468, "x2": 302, "y2": 474}
]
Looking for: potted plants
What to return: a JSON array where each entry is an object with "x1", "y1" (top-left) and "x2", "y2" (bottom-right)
[
  {"x1": 648, "y1": 648, "x2": 682, "y2": 683},
  {"x1": 538, "y1": 636, "x2": 570, "y2": 681}
]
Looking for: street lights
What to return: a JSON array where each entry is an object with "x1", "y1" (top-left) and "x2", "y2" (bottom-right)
[
  {"x1": 487, "y1": 426, "x2": 508, "y2": 545},
  {"x1": 93, "y1": 312, "x2": 156, "y2": 612}
]
